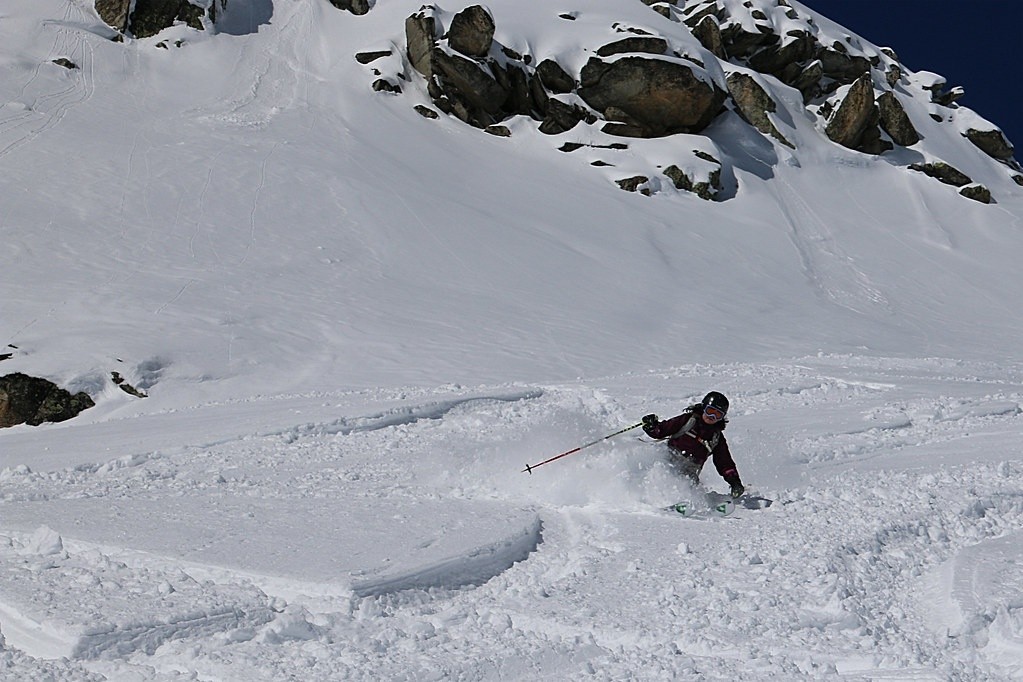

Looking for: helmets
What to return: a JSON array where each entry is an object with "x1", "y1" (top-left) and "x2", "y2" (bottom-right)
[{"x1": 702, "y1": 391, "x2": 729, "y2": 416}]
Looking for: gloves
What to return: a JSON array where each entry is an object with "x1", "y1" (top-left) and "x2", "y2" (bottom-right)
[
  {"x1": 730, "y1": 480, "x2": 744, "y2": 498},
  {"x1": 641, "y1": 414, "x2": 658, "y2": 433}
]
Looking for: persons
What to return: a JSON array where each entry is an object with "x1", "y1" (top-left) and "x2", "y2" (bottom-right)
[{"x1": 642, "y1": 391, "x2": 745, "y2": 499}]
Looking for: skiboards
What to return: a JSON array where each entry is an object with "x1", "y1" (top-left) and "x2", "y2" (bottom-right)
[{"x1": 663, "y1": 496, "x2": 736, "y2": 517}]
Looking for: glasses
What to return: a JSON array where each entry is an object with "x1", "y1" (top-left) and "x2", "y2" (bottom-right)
[{"x1": 703, "y1": 405, "x2": 725, "y2": 421}]
